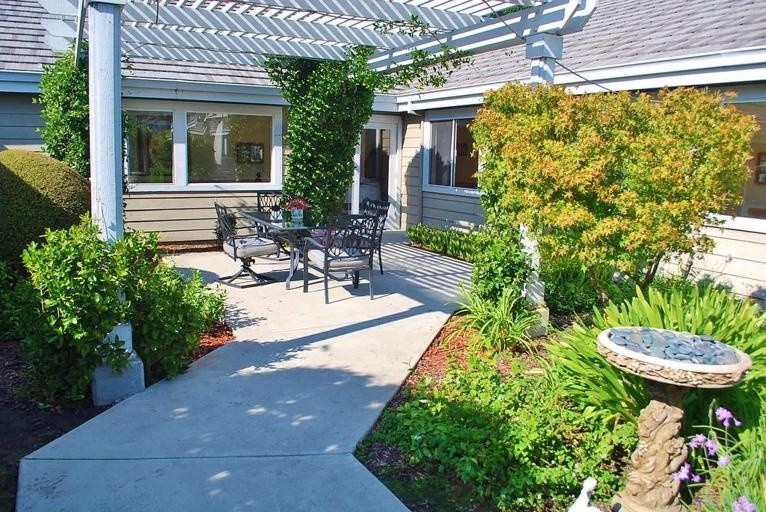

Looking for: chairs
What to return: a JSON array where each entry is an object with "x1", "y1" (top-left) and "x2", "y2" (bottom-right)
[{"x1": 213, "y1": 190, "x2": 391, "y2": 306}]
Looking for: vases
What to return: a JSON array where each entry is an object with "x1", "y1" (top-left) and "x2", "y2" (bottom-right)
[{"x1": 289, "y1": 207, "x2": 305, "y2": 223}]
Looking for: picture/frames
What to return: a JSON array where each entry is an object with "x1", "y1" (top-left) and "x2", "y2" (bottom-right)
[{"x1": 234, "y1": 142, "x2": 266, "y2": 165}]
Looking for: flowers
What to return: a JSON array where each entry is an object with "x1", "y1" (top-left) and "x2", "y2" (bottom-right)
[{"x1": 284, "y1": 196, "x2": 308, "y2": 211}]
